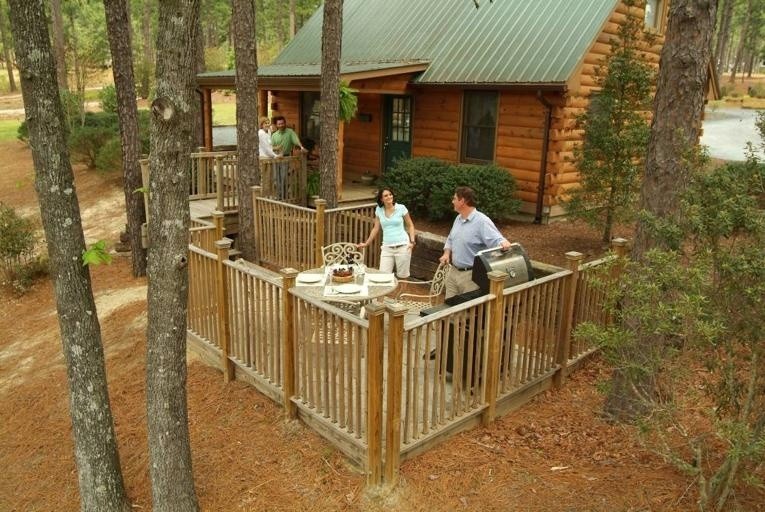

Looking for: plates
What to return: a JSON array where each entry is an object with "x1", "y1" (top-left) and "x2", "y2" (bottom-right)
[{"x1": 298, "y1": 265, "x2": 392, "y2": 294}]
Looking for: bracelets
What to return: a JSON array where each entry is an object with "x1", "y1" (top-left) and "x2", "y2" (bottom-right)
[
  {"x1": 410, "y1": 241, "x2": 415, "y2": 245},
  {"x1": 300, "y1": 146, "x2": 305, "y2": 149}
]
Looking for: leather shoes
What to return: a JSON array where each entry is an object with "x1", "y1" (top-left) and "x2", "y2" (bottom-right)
[{"x1": 423, "y1": 350, "x2": 435, "y2": 360}]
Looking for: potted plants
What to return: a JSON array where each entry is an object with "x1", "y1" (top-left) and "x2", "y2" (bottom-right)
[{"x1": 361, "y1": 170, "x2": 374, "y2": 186}]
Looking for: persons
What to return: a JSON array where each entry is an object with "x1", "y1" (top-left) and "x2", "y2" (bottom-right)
[
  {"x1": 257, "y1": 116, "x2": 284, "y2": 200},
  {"x1": 271, "y1": 115, "x2": 309, "y2": 201},
  {"x1": 422, "y1": 186, "x2": 511, "y2": 361},
  {"x1": 356, "y1": 187, "x2": 415, "y2": 301}
]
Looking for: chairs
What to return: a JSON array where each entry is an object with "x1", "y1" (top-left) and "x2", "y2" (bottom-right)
[{"x1": 234, "y1": 242, "x2": 452, "y2": 379}]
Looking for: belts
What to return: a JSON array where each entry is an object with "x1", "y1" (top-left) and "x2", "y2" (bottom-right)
[
  {"x1": 390, "y1": 245, "x2": 404, "y2": 248},
  {"x1": 452, "y1": 263, "x2": 472, "y2": 272}
]
